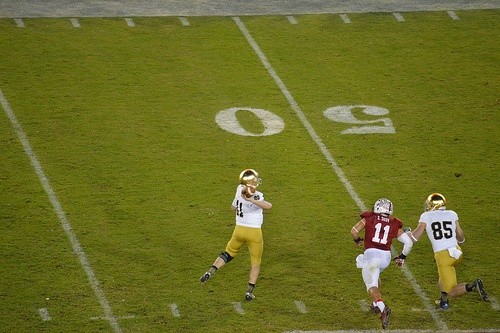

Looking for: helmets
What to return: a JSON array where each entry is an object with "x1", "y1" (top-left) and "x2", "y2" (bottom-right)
[
  {"x1": 424, "y1": 193, "x2": 447, "y2": 211},
  {"x1": 239, "y1": 169, "x2": 260, "y2": 188},
  {"x1": 374, "y1": 198, "x2": 394, "y2": 216}
]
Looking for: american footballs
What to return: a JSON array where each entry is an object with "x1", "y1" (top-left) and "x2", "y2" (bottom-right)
[{"x1": 241, "y1": 186, "x2": 255, "y2": 198}]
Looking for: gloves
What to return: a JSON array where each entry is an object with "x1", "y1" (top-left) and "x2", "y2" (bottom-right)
[
  {"x1": 354, "y1": 236, "x2": 364, "y2": 248},
  {"x1": 393, "y1": 256, "x2": 405, "y2": 267}
]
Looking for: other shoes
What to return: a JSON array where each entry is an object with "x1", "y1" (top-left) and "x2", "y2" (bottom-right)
[
  {"x1": 474, "y1": 277, "x2": 487, "y2": 301},
  {"x1": 200, "y1": 272, "x2": 211, "y2": 284},
  {"x1": 246, "y1": 292, "x2": 255, "y2": 301},
  {"x1": 380, "y1": 307, "x2": 391, "y2": 329},
  {"x1": 434, "y1": 297, "x2": 450, "y2": 309},
  {"x1": 370, "y1": 301, "x2": 381, "y2": 314}
]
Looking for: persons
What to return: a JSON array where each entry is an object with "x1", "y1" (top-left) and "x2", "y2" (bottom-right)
[
  {"x1": 200, "y1": 169, "x2": 272, "y2": 300},
  {"x1": 403, "y1": 192, "x2": 488, "y2": 310},
  {"x1": 351, "y1": 198, "x2": 414, "y2": 330}
]
